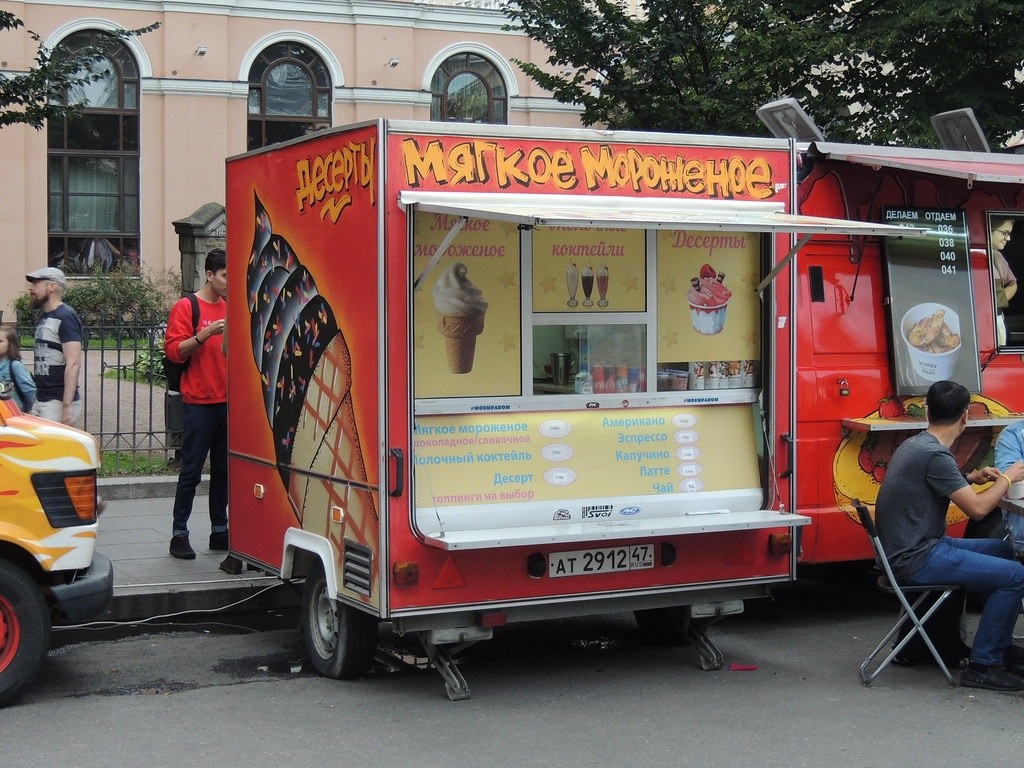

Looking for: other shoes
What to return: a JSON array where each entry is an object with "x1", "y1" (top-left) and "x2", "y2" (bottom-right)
[
  {"x1": 219, "y1": 555, "x2": 242, "y2": 574},
  {"x1": 1002, "y1": 643, "x2": 1024, "y2": 665},
  {"x1": 960, "y1": 661, "x2": 1024, "y2": 691}
]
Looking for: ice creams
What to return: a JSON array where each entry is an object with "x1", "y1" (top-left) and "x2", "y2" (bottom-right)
[
  {"x1": 432, "y1": 262, "x2": 488, "y2": 374},
  {"x1": 686, "y1": 263, "x2": 728, "y2": 337}
]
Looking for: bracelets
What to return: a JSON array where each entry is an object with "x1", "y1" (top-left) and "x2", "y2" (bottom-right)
[
  {"x1": 194, "y1": 334, "x2": 204, "y2": 344},
  {"x1": 999, "y1": 474, "x2": 1011, "y2": 490}
]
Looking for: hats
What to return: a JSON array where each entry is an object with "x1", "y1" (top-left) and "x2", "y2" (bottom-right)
[{"x1": 26, "y1": 267, "x2": 67, "y2": 292}]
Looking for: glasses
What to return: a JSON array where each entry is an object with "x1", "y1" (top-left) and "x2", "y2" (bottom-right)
[{"x1": 994, "y1": 228, "x2": 1012, "y2": 239}]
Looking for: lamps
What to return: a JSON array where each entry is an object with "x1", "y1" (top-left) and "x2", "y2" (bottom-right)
[
  {"x1": 196, "y1": 46, "x2": 206, "y2": 55},
  {"x1": 388, "y1": 57, "x2": 400, "y2": 67}
]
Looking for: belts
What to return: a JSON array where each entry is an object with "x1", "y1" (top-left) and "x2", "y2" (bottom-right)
[{"x1": 997, "y1": 307, "x2": 1002, "y2": 316}]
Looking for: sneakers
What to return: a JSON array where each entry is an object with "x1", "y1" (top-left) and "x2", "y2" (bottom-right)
[
  {"x1": 209, "y1": 529, "x2": 228, "y2": 551},
  {"x1": 169, "y1": 536, "x2": 196, "y2": 559}
]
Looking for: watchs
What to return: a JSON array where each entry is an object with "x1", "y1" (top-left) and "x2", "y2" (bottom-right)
[{"x1": 963, "y1": 473, "x2": 973, "y2": 485}]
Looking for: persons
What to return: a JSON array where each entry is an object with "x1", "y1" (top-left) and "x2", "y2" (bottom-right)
[
  {"x1": 991, "y1": 215, "x2": 1017, "y2": 345},
  {"x1": 995, "y1": 421, "x2": 1024, "y2": 568},
  {"x1": 165, "y1": 248, "x2": 227, "y2": 560},
  {"x1": 0, "y1": 325, "x2": 37, "y2": 413},
  {"x1": 875, "y1": 381, "x2": 1024, "y2": 692},
  {"x1": 25, "y1": 267, "x2": 106, "y2": 516}
]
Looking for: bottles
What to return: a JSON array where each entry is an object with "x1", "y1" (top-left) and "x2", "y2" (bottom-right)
[{"x1": 575, "y1": 362, "x2": 648, "y2": 395}]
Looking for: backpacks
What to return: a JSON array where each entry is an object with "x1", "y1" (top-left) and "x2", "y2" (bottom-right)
[
  {"x1": 162, "y1": 293, "x2": 200, "y2": 393},
  {"x1": 891, "y1": 583, "x2": 973, "y2": 670}
]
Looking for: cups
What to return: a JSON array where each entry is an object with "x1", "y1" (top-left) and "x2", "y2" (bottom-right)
[
  {"x1": 685, "y1": 297, "x2": 731, "y2": 335},
  {"x1": 550, "y1": 352, "x2": 571, "y2": 386},
  {"x1": 565, "y1": 271, "x2": 610, "y2": 308},
  {"x1": 901, "y1": 302, "x2": 961, "y2": 382}
]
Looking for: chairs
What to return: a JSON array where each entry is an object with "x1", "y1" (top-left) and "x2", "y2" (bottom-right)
[{"x1": 851, "y1": 498, "x2": 961, "y2": 689}]
